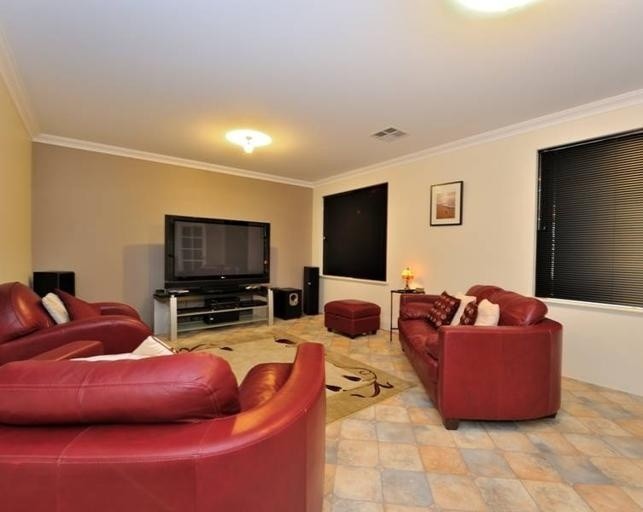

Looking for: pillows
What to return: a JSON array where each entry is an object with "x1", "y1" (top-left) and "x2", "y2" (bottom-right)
[
  {"x1": 425, "y1": 290, "x2": 500, "y2": 332},
  {"x1": 39, "y1": 290, "x2": 68, "y2": 324},
  {"x1": 55, "y1": 288, "x2": 100, "y2": 320},
  {"x1": 70, "y1": 333, "x2": 175, "y2": 361}
]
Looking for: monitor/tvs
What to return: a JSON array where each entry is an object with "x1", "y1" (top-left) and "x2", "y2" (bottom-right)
[{"x1": 165, "y1": 214, "x2": 270, "y2": 293}]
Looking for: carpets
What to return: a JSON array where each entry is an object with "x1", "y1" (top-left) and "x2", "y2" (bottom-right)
[{"x1": 165, "y1": 330, "x2": 418, "y2": 428}]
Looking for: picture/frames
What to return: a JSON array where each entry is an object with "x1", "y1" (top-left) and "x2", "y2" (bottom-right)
[{"x1": 429, "y1": 181, "x2": 462, "y2": 227}]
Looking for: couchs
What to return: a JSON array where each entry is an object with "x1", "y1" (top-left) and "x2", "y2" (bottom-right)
[
  {"x1": 394, "y1": 281, "x2": 564, "y2": 432},
  {"x1": 0, "y1": 332, "x2": 329, "y2": 511},
  {"x1": 1, "y1": 279, "x2": 150, "y2": 360},
  {"x1": 322, "y1": 298, "x2": 381, "y2": 339}
]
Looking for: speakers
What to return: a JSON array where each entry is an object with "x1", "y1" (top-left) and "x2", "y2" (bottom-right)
[
  {"x1": 273, "y1": 287, "x2": 302, "y2": 320},
  {"x1": 304, "y1": 266, "x2": 319, "y2": 315},
  {"x1": 33, "y1": 271, "x2": 75, "y2": 299}
]
